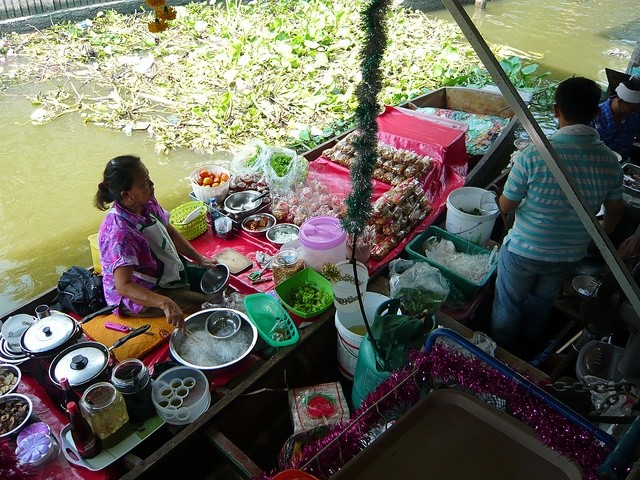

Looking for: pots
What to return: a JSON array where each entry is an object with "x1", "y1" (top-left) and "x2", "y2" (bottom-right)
[
  {"x1": 48, "y1": 324, "x2": 152, "y2": 388},
  {"x1": 20, "y1": 315, "x2": 81, "y2": 357},
  {"x1": 223, "y1": 190, "x2": 263, "y2": 215}
]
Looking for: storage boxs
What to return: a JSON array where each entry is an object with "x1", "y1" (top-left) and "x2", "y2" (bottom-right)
[{"x1": 405, "y1": 225, "x2": 497, "y2": 299}]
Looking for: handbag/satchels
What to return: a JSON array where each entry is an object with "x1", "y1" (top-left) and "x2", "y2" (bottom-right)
[{"x1": 56, "y1": 266, "x2": 107, "y2": 317}]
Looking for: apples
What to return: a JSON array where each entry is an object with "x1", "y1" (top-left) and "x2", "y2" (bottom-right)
[{"x1": 194, "y1": 170, "x2": 229, "y2": 188}]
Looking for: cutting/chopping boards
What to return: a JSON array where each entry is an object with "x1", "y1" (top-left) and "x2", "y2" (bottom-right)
[
  {"x1": 82, "y1": 312, "x2": 192, "y2": 361},
  {"x1": 212, "y1": 248, "x2": 253, "y2": 275}
]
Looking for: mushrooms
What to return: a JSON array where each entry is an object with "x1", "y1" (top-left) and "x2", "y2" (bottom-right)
[{"x1": 0, "y1": 400, "x2": 29, "y2": 435}]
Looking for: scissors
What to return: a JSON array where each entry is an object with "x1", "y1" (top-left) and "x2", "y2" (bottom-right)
[{"x1": 248, "y1": 261, "x2": 271, "y2": 280}]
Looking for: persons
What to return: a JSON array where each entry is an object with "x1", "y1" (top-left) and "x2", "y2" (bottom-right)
[
  {"x1": 590, "y1": 78, "x2": 640, "y2": 162},
  {"x1": 95, "y1": 154, "x2": 223, "y2": 333},
  {"x1": 490, "y1": 76, "x2": 624, "y2": 370}
]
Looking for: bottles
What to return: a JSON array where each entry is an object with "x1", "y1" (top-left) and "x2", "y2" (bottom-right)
[
  {"x1": 66, "y1": 401, "x2": 97, "y2": 459},
  {"x1": 60, "y1": 378, "x2": 79, "y2": 420}
]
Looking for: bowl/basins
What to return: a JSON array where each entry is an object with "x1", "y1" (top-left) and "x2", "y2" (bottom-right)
[
  {"x1": 204, "y1": 310, "x2": 242, "y2": 339},
  {"x1": 266, "y1": 223, "x2": 300, "y2": 245},
  {"x1": 169, "y1": 308, "x2": 258, "y2": 370},
  {"x1": 200, "y1": 264, "x2": 229, "y2": 295},
  {"x1": 0, "y1": 393, "x2": 32, "y2": 437},
  {"x1": 0, "y1": 315, "x2": 27, "y2": 362},
  {"x1": 240, "y1": 213, "x2": 276, "y2": 234},
  {"x1": 1, "y1": 364, "x2": 21, "y2": 396},
  {"x1": 570, "y1": 274, "x2": 606, "y2": 299}
]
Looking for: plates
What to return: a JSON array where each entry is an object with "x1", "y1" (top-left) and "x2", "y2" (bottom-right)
[
  {"x1": 60, "y1": 422, "x2": 165, "y2": 472},
  {"x1": 621, "y1": 163, "x2": 640, "y2": 193},
  {"x1": 514, "y1": 137, "x2": 532, "y2": 150},
  {"x1": 323, "y1": 387, "x2": 519, "y2": 478}
]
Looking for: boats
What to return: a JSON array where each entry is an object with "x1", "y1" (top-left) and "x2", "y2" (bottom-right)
[
  {"x1": 207, "y1": 163, "x2": 640, "y2": 480},
  {"x1": 0, "y1": 86, "x2": 518, "y2": 480}
]
